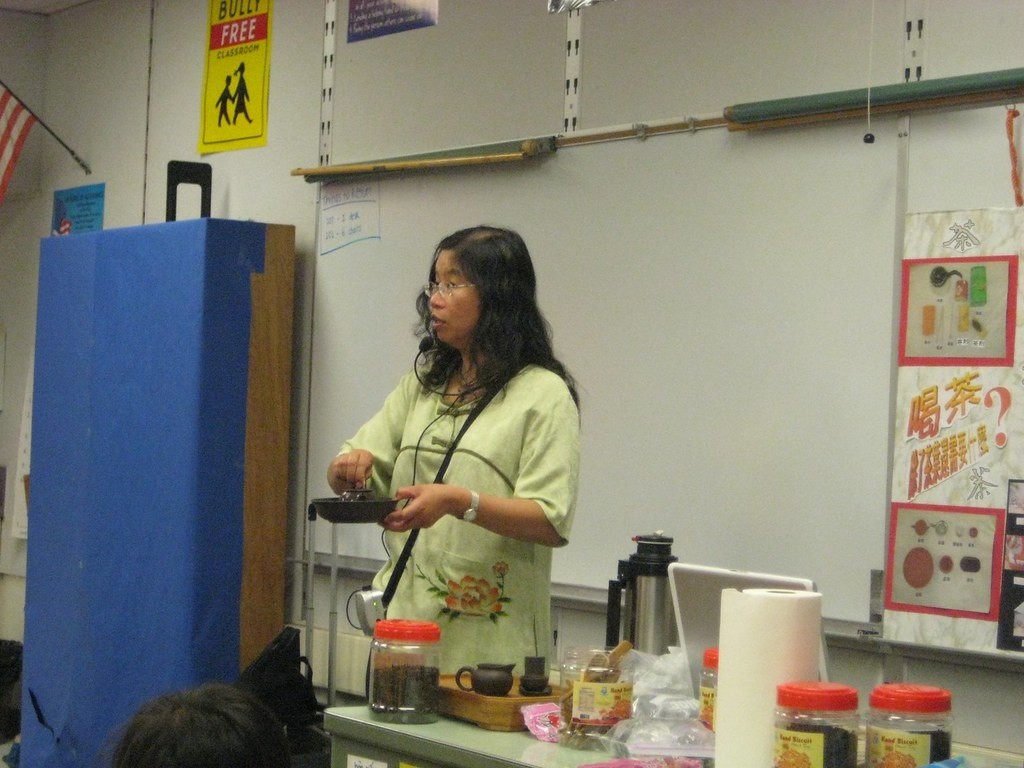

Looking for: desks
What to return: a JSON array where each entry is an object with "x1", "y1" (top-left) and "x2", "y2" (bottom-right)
[{"x1": 325, "y1": 701, "x2": 1023, "y2": 768}]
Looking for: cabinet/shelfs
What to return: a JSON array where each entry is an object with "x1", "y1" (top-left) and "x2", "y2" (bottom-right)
[{"x1": 19, "y1": 217, "x2": 296, "y2": 768}]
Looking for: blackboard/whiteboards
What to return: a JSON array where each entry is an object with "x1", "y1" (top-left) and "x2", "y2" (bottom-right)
[{"x1": 291, "y1": 85, "x2": 1024, "y2": 675}]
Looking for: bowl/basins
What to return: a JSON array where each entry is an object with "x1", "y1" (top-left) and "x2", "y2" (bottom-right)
[{"x1": 312, "y1": 498, "x2": 399, "y2": 524}]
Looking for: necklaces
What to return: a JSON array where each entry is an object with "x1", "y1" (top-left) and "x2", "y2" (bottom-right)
[{"x1": 455, "y1": 368, "x2": 479, "y2": 402}]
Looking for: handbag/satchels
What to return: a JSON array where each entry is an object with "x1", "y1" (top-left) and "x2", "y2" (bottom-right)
[{"x1": 237, "y1": 625, "x2": 302, "y2": 697}]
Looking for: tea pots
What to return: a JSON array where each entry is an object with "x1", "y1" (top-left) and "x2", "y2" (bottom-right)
[
  {"x1": 342, "y1": 485, "x2": 375, "y2": 501},
  {"x1": 930, "y1": 519, "x2": 949, "y2": 535}
]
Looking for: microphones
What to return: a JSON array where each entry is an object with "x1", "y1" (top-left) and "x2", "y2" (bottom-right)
[{"x1": 414, "y1": 336, "x2": 483, "y2": 395}]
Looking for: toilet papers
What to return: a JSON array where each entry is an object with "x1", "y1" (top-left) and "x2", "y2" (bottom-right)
[{"x1": 713, "y1": 586, "x2": 827, "y2": 768}]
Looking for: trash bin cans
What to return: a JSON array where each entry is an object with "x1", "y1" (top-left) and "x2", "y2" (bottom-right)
[{"x1": 240, "y1": 628, "x2": 336, "y2": 768}]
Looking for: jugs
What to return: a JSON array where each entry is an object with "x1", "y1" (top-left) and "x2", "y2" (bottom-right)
[{"x1": 605, "y1": 530, "x2": 678, "y2": 656}]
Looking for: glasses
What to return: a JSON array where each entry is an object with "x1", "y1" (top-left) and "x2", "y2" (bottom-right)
[{"x1": 423, "y1": 280, "x2": 477, "y2": 299}]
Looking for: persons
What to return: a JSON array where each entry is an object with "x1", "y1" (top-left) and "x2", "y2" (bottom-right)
[
  {"x1": 0, "y1": 638, "x2": 26, "y2": 768},
  {"x1": 328, "y1": 224, "x2": 580, "y2": 678},
  {"x1": 105, "y1": 684, "x2": 295, "y2": 768}
]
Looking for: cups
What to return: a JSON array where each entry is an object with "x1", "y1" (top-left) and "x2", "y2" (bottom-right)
[
  {"x1": 921, "y1": 305, "x2": 935, "y2": 335},
  {"x1": 455, "y1": 662, "x2": 516, "y2": 697}
]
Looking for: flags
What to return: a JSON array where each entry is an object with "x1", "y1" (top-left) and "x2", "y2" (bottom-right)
[{"x1": 0, "y1": 81, "x2": 38, "y2": 205}]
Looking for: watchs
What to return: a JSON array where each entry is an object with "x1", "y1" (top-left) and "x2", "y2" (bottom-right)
[{"x1": 464, "y1": 490, "x2": 480, "y2": 522}]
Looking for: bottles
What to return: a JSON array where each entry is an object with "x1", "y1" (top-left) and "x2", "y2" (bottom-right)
[
  {"x1": 561, "y1": 648, "x2": 635, "y2": 749},
  {"x1": 368, "y1": 620, "x2": 442, "y2": 724},
  {"x1": 699, "y1": 648, "x2": 719, "y2": 732},
  {"x1": 772, "y1": 683, "x2": 860, "y2": 767},
  {"x1": 866, "y1": 683, "x2": 953, "y2": 766}
]
